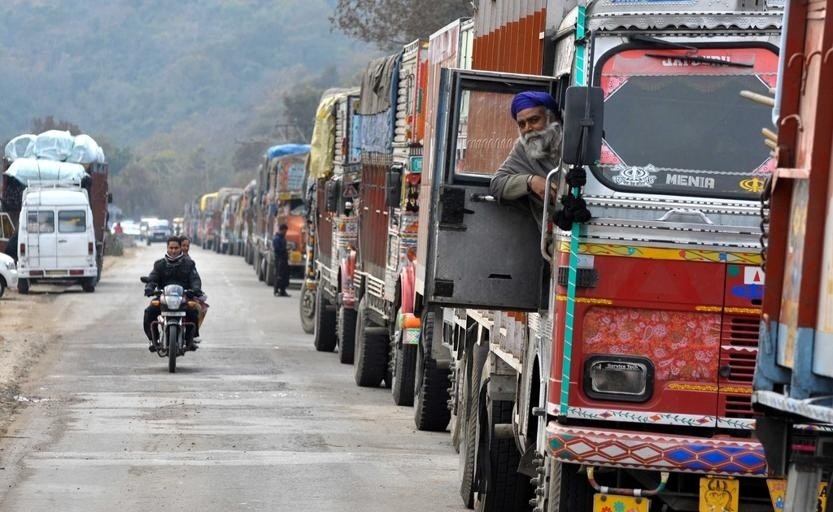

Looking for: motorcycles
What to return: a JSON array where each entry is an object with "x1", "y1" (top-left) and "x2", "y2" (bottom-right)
[{"x1": 139, "y1": 277, "x2": 201, "y2": 371}]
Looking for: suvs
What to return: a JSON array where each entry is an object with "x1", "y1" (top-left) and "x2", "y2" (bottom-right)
[{"x1": 145, "y1": 220, "x2": 171, "y2": 245}]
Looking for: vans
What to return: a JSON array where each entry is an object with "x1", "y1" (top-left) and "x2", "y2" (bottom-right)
[
  {"x1": 14, "y1": 185, "x2": 98, "y2": 292},
  {"x1": 138, "y1": 218, "x2": 158, "y2": 240}
]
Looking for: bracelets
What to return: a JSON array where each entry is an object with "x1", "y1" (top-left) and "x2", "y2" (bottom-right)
[{"x1": 527, "y1": 174, "x2": 535, "y2": 188}]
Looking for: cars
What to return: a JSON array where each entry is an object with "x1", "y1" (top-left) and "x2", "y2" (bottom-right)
[{"x1": 0, "y1": 255, "x2": 17, "y2": 298}]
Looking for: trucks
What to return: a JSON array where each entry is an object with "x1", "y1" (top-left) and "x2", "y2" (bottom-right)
[{"x1": 0, "y1": 160, "x2": 112, "y2": 281}]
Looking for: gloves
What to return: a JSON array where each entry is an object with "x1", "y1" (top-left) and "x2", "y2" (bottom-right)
[
  {"x1": 194, "y1": 288, "x2": 202, "y2": 297},
  {"x1": 145, "y1": 286, "x2": 153, "y2": 296}
]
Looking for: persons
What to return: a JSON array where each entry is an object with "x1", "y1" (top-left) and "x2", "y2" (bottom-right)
[
  {"x1": 113, "y1": 222, "x2": 123, "y2": 234},
  {"x1": 488, "y1": 90, "x2": 563, "y2": 247},
  {"x1": 177, "y1": 235, "x2": 192, "y2": 258},
  {"x1": 143, "y1": 236, "x2": 202, "y2": 353},
  {"x1": 4, "y1": 228, "x2": 18, "y2": 265},
  {"x1": 272, "y1": 223, "x2": 292, "y2": 297}
]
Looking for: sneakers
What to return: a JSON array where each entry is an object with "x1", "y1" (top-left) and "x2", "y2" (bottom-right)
[
  {"x1": 274, "y1": 290, "x2": 291, "y2": 296},
  {"x1": 194, "y1": 336, "x2": 201, "y2": 342}
]
